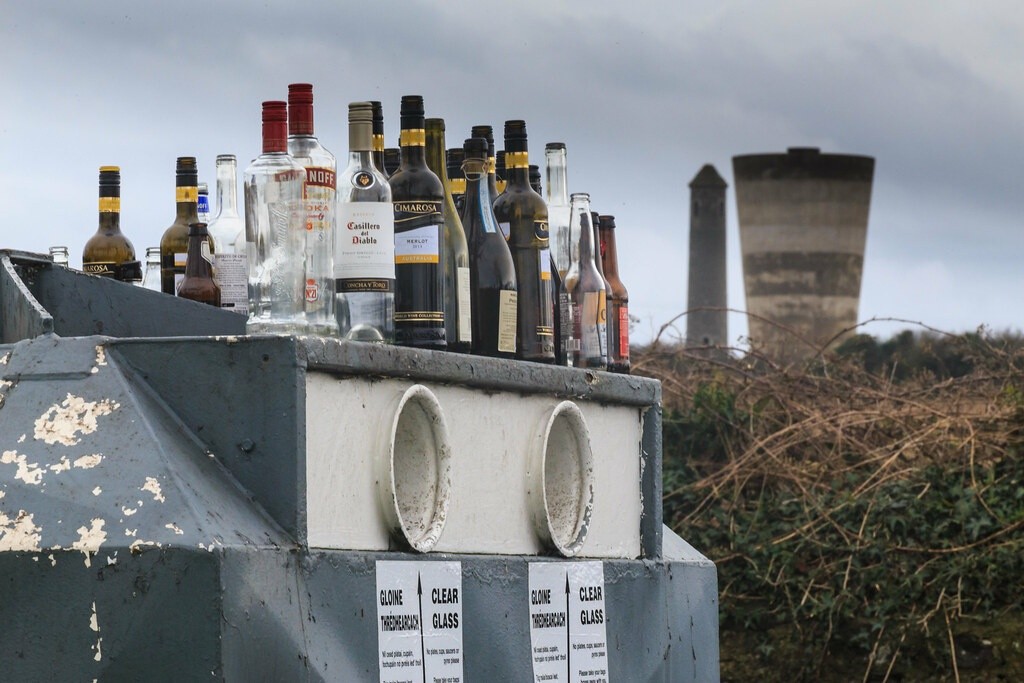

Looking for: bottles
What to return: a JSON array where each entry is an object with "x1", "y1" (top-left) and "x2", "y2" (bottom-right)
[
  {"x1": 120, "y1": 83, "x2": 632, "y2": 375},
  {"x1": 48, "y1": 246, "x2": 68, "y2": 267},
  {"x1": 82, "y1": 166, "x2": 136, "y2": 281}
]
